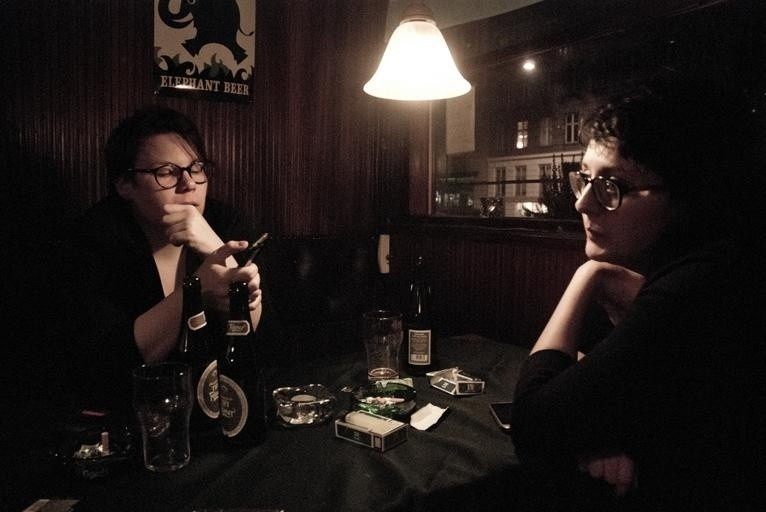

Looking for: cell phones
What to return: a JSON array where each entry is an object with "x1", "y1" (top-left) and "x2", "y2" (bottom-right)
[
  {"x1": 488, "y1": 401, "x2": 512, "y2": 433},
  {"x1": 239, "y1": 232, "x2": 268, "y2": 267}
]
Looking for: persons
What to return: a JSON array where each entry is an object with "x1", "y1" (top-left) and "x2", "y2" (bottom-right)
[
  {"x1": 508, "y1": 76, "x2": 765, "y2": 512},
  {"x1": 54, "y1": 105, "x2": 268, "y2": 422}
]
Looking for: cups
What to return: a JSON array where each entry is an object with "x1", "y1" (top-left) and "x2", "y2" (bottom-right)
[
  {"x1": 134, "y1": 363, "x2": 193, "y2": 472},
  {"x1": 362, "y1": 311, "x2": 404, "y2": 379}
]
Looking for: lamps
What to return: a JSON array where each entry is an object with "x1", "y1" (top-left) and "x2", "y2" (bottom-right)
[{"x1": 361, "y1": 0, "x2": 471, "y2": 99}]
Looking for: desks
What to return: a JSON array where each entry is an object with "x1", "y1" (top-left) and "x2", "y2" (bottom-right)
[{"x1": 0, "y1": 332, "x2": 534, "y2": 510}]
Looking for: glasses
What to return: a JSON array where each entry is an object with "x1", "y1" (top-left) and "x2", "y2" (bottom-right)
[
  {"x1": 567, "y1": 167, "x2": 656, "y2": 209},
  {"x1": 136, "y1": 155, "x2": 215, "y2": 191}
]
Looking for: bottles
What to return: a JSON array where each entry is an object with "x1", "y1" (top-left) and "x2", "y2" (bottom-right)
[
  {"x1": 176, "y1": 277, "x2": 218, "y2": 448},
  {"x1": 405, "y1": 256, "x2": 436, "y2": 376},
  {"x1": 219, "y1": 283, "x2": 268, "y2": 446}
]
